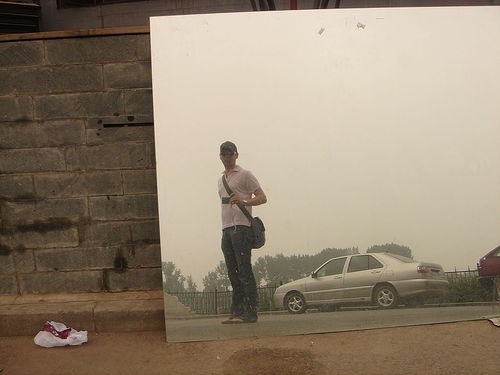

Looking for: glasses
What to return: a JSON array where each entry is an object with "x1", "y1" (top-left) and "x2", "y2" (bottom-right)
[{"x1": 221, "y1": 151, "x2": 236, "y2": 156}]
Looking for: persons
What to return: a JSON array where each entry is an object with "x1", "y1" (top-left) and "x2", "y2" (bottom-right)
[{"x1": 218, "y1": 140, "x2": 267, "y2": 324}]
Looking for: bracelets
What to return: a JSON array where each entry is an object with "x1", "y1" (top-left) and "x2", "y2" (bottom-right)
[{"x1": 242, "y1": 199, "x2": 247, "y2": 205}]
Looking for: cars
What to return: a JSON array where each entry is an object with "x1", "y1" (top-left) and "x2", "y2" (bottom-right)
[
  {"x1": 274, "y1": 252, "x2": 450, "y2": 315},
  {"x1": 477, "y1": 246, "x2": 500, "y2": 300}
]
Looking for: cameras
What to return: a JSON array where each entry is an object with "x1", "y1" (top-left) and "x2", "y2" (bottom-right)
[{"x1": 222, "y1": 197, "x2": 231, "y2": 204}]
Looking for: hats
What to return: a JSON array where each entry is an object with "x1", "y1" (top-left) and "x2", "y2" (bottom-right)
[{"x1": 220, "y1": 141, "x2": 237, "y2": 154}]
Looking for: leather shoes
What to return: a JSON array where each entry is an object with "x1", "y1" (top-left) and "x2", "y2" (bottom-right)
[{"x1": 222, "y1": 316, "x2": 257, "y2": 324}]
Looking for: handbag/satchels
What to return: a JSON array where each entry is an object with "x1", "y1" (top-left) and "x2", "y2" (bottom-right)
[{"x1": 249, "y1": 217, "x2": 265, "y2": 249}]
